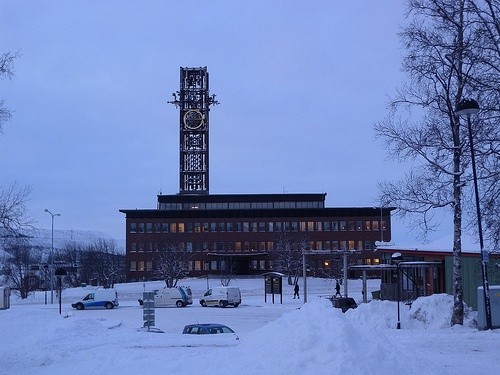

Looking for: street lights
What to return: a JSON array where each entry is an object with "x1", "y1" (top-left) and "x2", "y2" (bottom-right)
[
  {"x1": 380, "y1": 200, "x2": 392, "y2": 242},
  {"x1": 44, "y1": 209, "x2": 61, "y2": 304},
  {"x1": 392, "y1": 252, "x2": 403, "y2": 329},
  {"x1": 55, "y1": 268, "x2": 67, "y2": 315},
  {"x1": 455, "y1": 98, "x2": 493, "y2": 331}
]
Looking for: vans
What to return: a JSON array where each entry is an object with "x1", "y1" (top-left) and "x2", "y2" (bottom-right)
[
  {"x1": 199, "y1": 287, "x2": 242, "y2": 308},
  {"x1": 71, "y1": 288, "x2": 120, "y2": 310},
  {"x1": 138, "y1": 286, "x2": 193, "y2": 308},
  {"x1": 182, "y1": 323, "x2": 240, "y2": 347}
]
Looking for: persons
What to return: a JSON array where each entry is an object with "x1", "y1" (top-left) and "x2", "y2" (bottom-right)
[
  {"x1": 293, "y1": 282, "x2": 299, "y2": 299},
  {"x1": 335, "y1": 279, "x2": 342, "y2": 298}
]
